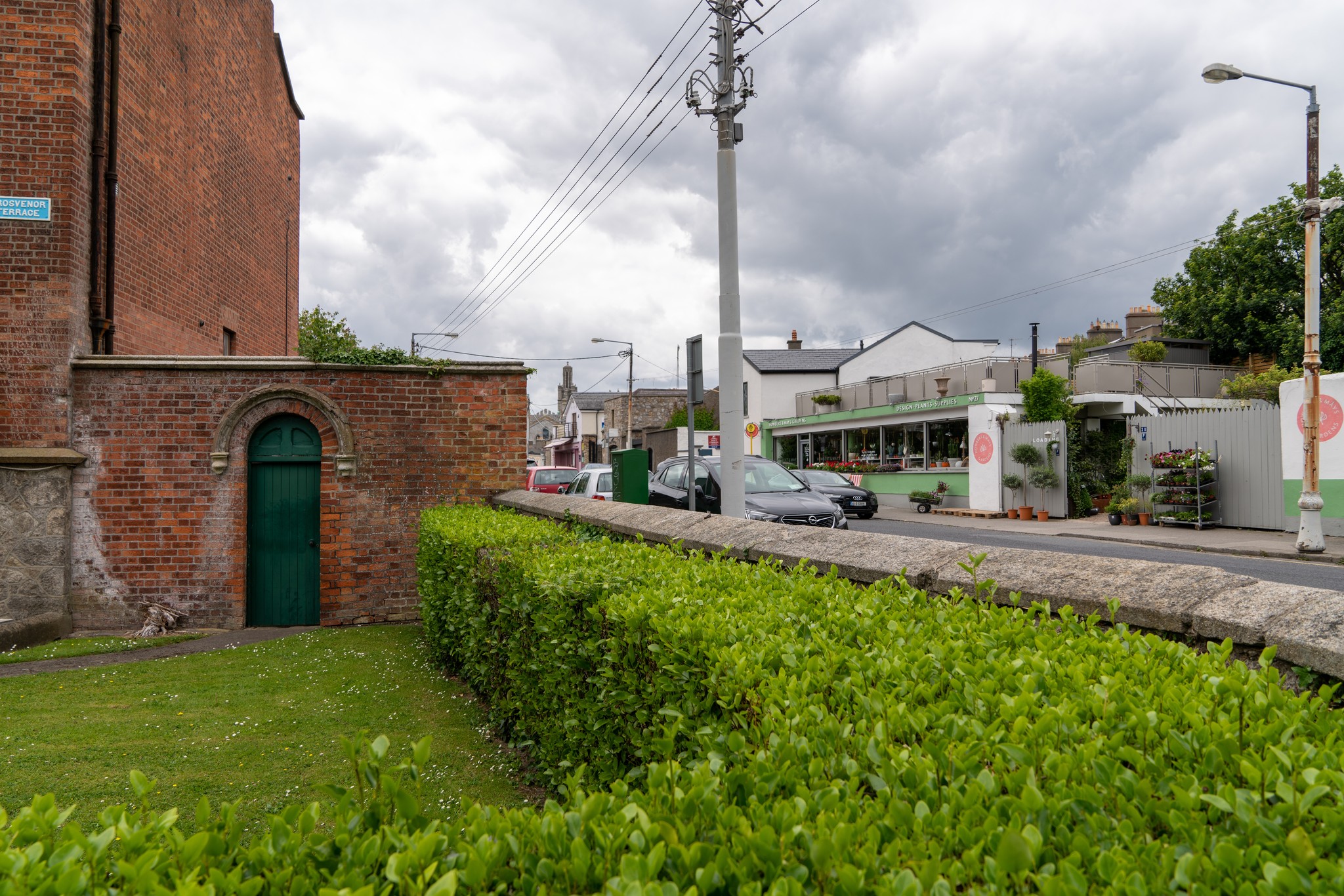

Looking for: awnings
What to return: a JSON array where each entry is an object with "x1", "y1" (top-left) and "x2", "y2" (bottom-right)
[{"x1": 544, "y1": 439, "x2": 573, "y2": 448}]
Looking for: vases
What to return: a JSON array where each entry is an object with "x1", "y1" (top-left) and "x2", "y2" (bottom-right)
[
  {"x1": 937, "y1": 489, "x2": 944, "y2": 493},
  {"x1": 1153, "y1": 464, "x2": 1209, "y2": 522}
]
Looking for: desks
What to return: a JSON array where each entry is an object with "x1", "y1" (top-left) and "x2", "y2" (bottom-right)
[
  {"x1": 886, "y1": 457, "x2": 924, "y2": 468},
  {"x1": 851, "y1": 459, "x2": 880, "y2": 465}
]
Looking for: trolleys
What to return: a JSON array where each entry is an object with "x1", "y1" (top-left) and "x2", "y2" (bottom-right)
[{"x1": 907, "y1": 485, "x2": 950, "y2": 515}]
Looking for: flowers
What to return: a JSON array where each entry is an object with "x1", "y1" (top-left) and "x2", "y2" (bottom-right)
[
  {"x1": 805, "y1": 461, "x2": 903, "y2": 473},
  {"x1": 1145, "y1": 447, "x2": 1217, "y2": 503},
  {"x1": 910, "y1": 480, "x2": 950, "y2": 500}
]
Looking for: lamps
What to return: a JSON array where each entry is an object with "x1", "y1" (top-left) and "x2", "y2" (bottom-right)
[
  {"x1": 936, "y1": 397, "x2": 941, "y2": 400},
  {"x1": 889, "y1": 402, "x2": 898, "y2": 406},
  {"x1": 945, "y1": 422, "x2": 952, "y2": 436},
  {"x1": 794, "y1": 416, "x2": 798, "y2": 419},
  {"x1": 960, "y1": 435, "x2": 967, "y2": 461},
  {"x1": 815, "y1": 412, "x2": 821, "y2": 416},
  {"x1": 775, "y1": 419, "x2": 778, "y2": 421},
  {"x1": 849, "y1": 408, "x2": 855, "y2": 412}
]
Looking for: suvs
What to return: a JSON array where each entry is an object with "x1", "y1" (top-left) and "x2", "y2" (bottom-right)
[{"x1": 647, "y1": 454, "x2": 849, "y2": 530}]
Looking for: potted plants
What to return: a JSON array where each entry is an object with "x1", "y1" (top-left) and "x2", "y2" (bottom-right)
[
  {"x1": 847, "y1": 438, "x2": 961, "y2": 468},
  {"x1": 811, "y1": 394, "x2": 842, "y2": 413},
  {"x1": 1001, "y1": 427, "x2": 1186, "y2": 526}
]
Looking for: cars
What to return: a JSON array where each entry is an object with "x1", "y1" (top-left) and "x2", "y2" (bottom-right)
[
  {"x1": 581, "y1": 463, "x2": 613, "y2": 470},
  {"x1": 526, "y1": 466, "x2": 581, "y2": 495},
  {"x1": 556, "y1": 469, "x2": 614, "y2": 501},
  {"x1": 527, "y1": 458, "x2": 538, "y2": 468},
  {"x1": 769, "y1": 471, "x2": 879, "y2": 520}
]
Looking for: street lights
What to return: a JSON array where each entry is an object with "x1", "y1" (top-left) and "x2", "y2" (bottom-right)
[
  {"x1": 411, "y1": 333, "x2": 459, "y2": 357},
  {"x1": 591, "y1": 338, "x2": 633, "y2": 448},
  {"x1": 1201, "y1": 62, "x2": 1327, "y2": 552}
]
